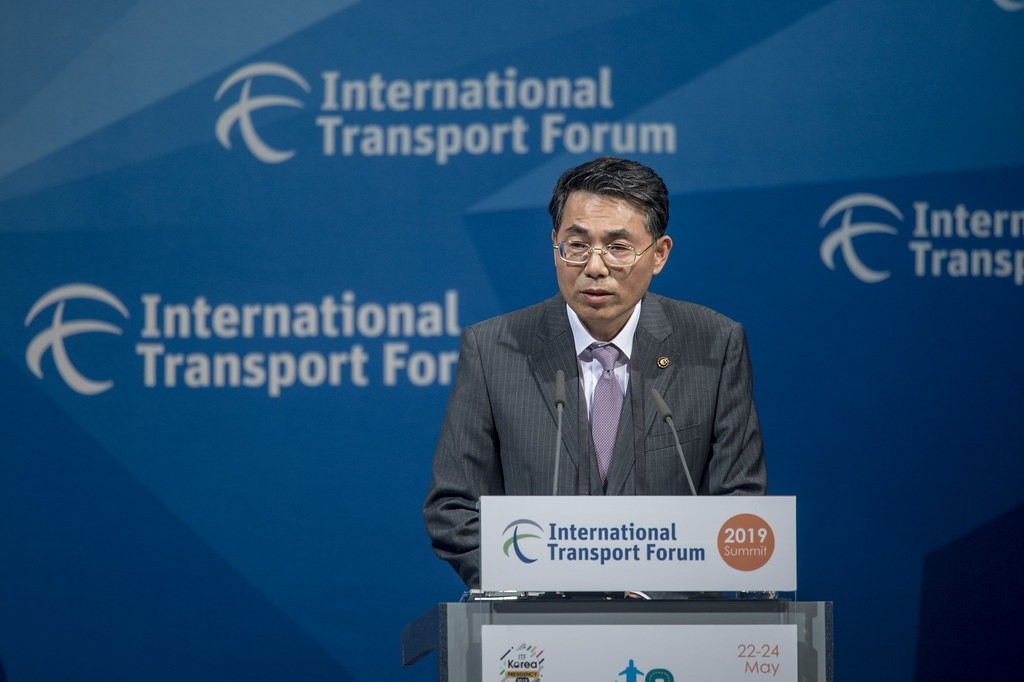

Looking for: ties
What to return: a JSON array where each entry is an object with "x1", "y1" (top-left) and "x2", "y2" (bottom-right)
[{"x1": 585, "y1": 344, "x2": 624, "y2": 488}]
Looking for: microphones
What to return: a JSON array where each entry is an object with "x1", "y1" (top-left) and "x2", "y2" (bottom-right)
[
  {"x1": 651, "y1": 388, "x2": 697, "y2": 496},
  {"x1": 552, "y1": 370, "x2": 567, "y2": 495}
]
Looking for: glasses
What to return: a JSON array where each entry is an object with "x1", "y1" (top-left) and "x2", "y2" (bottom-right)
[{"x1": 552, "y1": 230, "x2": 658, "y2": 267}]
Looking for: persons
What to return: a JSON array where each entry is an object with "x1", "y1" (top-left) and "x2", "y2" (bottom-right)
[{"x1": 424, "y1": 158, "x2": 767, "y2": 589}]
